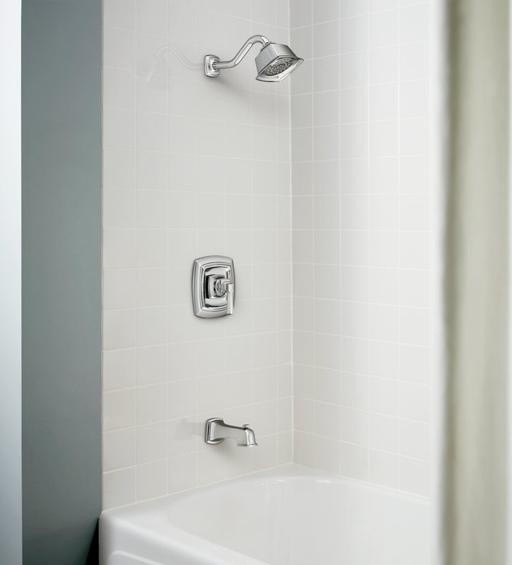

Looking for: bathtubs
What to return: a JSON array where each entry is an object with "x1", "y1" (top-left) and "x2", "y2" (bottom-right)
[{"x1": 96, "y1": 461, "x2": 441, "y2": 565}]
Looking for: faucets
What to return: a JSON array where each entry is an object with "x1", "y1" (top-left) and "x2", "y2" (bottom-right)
[{"x1": 203, "y1": 417, "x2": 257, "y2": 450}]
